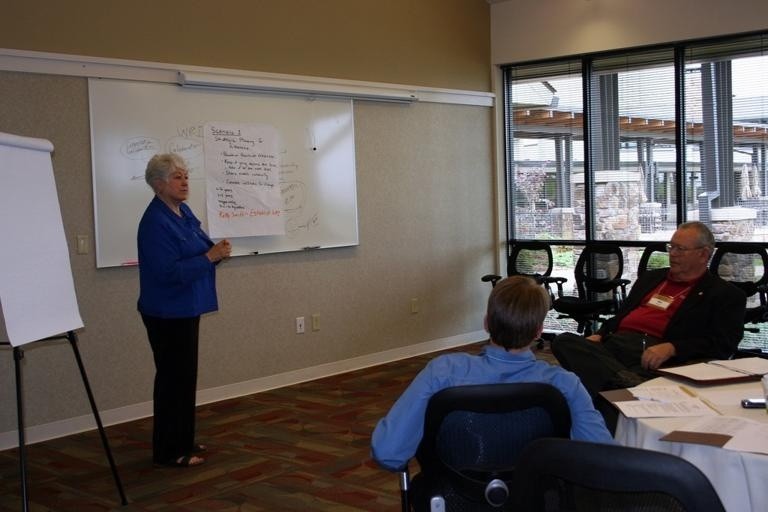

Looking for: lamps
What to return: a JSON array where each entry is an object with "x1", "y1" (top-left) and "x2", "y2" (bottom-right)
[{"x1": 176, "y1": 72, "x2": 420, "y2": 104}]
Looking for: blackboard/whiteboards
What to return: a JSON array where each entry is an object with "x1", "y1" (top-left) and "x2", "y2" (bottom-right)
[{"x1": 85, "y1": 77, "x2": 359, "y2": 269}]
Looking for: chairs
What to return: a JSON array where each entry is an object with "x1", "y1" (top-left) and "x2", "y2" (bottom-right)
[{"x1": 482, "y1": 243, "x2": 768, "y2": 360}]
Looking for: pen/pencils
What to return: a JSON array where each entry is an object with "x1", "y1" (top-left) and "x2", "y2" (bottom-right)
[
  {"x1": 700, "y1": 399, "x2": 725, "y2": 416},
  {"x1": 680, "y1": 384, "x2": 697, "y2": 397}
]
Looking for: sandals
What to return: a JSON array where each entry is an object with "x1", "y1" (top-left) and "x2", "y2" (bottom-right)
[{"x1": 154, "y1": 444, "x2": 207, "y2": 468}]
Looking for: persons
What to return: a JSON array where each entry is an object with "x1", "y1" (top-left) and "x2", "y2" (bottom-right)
[
  {"x1": 368, "y1": 271, "x2": 625, "y2": 512},
  {"x1": 135, "y1": 153, "x2": 233, "y2": 467},
  {"x1": 550, "y1": 219, "x2": 747, "y2": 443}
]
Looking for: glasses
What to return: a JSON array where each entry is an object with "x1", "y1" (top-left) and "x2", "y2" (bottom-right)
[{"x1": 666, "y1": 243, "x2": 707, "y2": 254}]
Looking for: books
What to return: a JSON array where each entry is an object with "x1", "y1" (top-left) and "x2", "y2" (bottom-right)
[{"x1": 652, "y1": 356, "x2": 768, "y2": 388}]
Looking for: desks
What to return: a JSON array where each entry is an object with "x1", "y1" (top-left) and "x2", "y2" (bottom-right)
[{"x1": 614, "y1": 357, "x2": 768, "y2": 511}]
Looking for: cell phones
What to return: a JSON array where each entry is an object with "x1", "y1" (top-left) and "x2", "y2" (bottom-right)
[{"x1": 742, "y1": 399, "x2": 766, "y2": 408}]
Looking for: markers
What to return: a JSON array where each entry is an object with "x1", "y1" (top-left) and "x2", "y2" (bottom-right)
[{"x1": 303, "y1": 245, "x2": 320, "y2": 250}]
